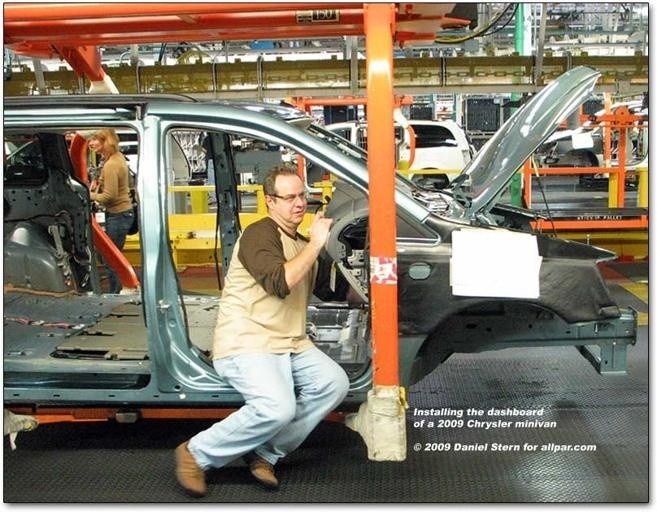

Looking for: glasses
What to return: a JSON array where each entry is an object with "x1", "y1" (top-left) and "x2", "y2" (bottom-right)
[{"x1": 271, "y1": 193, "x2": 307, "y2": 201}]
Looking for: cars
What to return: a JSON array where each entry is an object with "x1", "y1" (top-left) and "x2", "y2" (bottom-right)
[
  {"x1": 109, "y1": 126, "x2": 142, "y2": 178},
  {"x1": 539, "y1": 101, "x2": 648, "y2": 188}
]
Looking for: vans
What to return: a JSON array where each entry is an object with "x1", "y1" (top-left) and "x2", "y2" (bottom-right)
[
  {"x1": 317, "y1": 114, "x2": 476, "y2": 194},
  {"x1": 2, "y1": 66, "x2": 640, "y2": 413}
]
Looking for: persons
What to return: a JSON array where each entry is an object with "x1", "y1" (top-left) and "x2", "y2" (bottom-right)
[
  {"x1": 176, "y1": 168, "x2": 363, "y2": 497},
  {"x1": 89, "y1": 128, "x2": 134, "y2": 292}
]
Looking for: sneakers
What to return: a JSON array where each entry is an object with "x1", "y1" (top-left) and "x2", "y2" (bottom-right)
[
  {"x1": 174, "y1": 441, "x2": 207, "y2": 496},
  {"x1": 243, "y1": 450, "x2": 278, "y2": 489}
]
